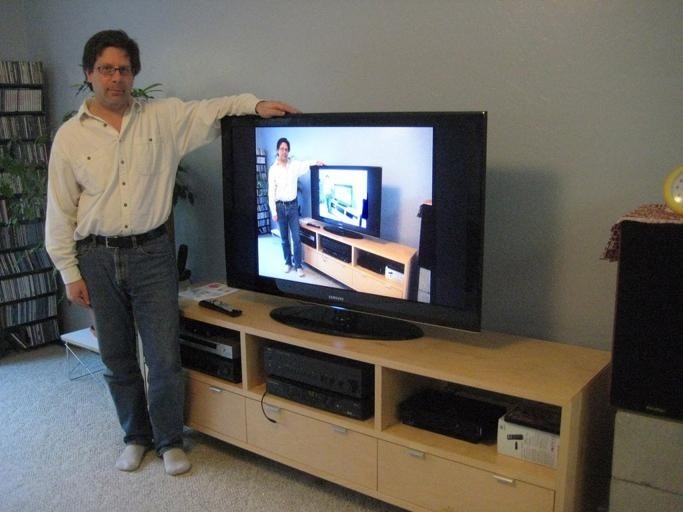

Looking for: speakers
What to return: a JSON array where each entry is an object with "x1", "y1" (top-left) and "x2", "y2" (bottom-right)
[{"x1": 610, "y1": 219, "x2": 682, "y2": 421}]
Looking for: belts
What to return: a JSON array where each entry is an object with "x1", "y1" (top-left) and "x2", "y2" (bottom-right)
[
  {"x1": 276, "y1": 200, "x2": 296, "y2": 204},
  {"x1": 86, "y1": 225, "x2": 165, "y2": 248}
]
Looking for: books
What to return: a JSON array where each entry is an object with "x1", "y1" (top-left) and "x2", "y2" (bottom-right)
[
  {"x1": 256, "y1": 147, "x2": 270, "y2": 235},
  {"x1": 178, "y1": 281, "x2": 239, "y2": 304},
  {"x1": 0, "y1": 59, "x2": 61, "y2": 351}
]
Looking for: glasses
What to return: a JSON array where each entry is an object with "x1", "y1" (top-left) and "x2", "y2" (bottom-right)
[{"x1": 94, "y1": 64, "x2": 133, "y2": 75}]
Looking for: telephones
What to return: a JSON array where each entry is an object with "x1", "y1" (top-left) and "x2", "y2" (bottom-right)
[
  {"x1": 177, "y1": 243, "x2": 192, "y2": 280},
  {"x1": 298, "y1": 205, "x2": 302, "y2": 216}
]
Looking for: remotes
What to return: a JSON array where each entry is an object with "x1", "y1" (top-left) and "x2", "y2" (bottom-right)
[
  {"x1": 307, "y1": 222, "x2": 321, "y2": 229},
  {"x1": 200, "y1": 298, "x2": 242, "y2": 317}
]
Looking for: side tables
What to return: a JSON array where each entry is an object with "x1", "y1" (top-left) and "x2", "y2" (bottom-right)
[{"x1": 60, "y1": 326, "x2": 108, "y2": 389}]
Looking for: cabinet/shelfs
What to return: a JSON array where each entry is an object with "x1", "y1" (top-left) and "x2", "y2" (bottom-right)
[
  {"x1": 290, "y1": 218, "x2": 419, "y2": 302},
  {"x1": 0, "y1": 82, "x2": 66, "y2": 360},
  {"x1": 255, "y1": 156, "x2": 271, "y2": 236},
  {"x1": 176, "y1": 278, "x2": 615, "y2": 511}
]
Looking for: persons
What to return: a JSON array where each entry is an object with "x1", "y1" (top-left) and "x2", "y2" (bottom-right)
[
  {"x1": 44, "y1": 29, "x2": 302, "y2": 476},
  {"x1": 324, "y1": 174, "x2": 336, "y2": 214},
  {"x1": 266, "y1": 138, "x2": 328, "y2": 276}
]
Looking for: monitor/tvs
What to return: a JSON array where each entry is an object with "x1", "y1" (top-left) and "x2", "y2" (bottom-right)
[
  {"x1": 333, "y1": 183, "x2": 358, "y2": 206},
  {"x1": 309, "y1": 165, "x2": 382, "y2": 239},
  {"x1": 221, "y1": 110, "x2": 487, "y2": 340}
]
